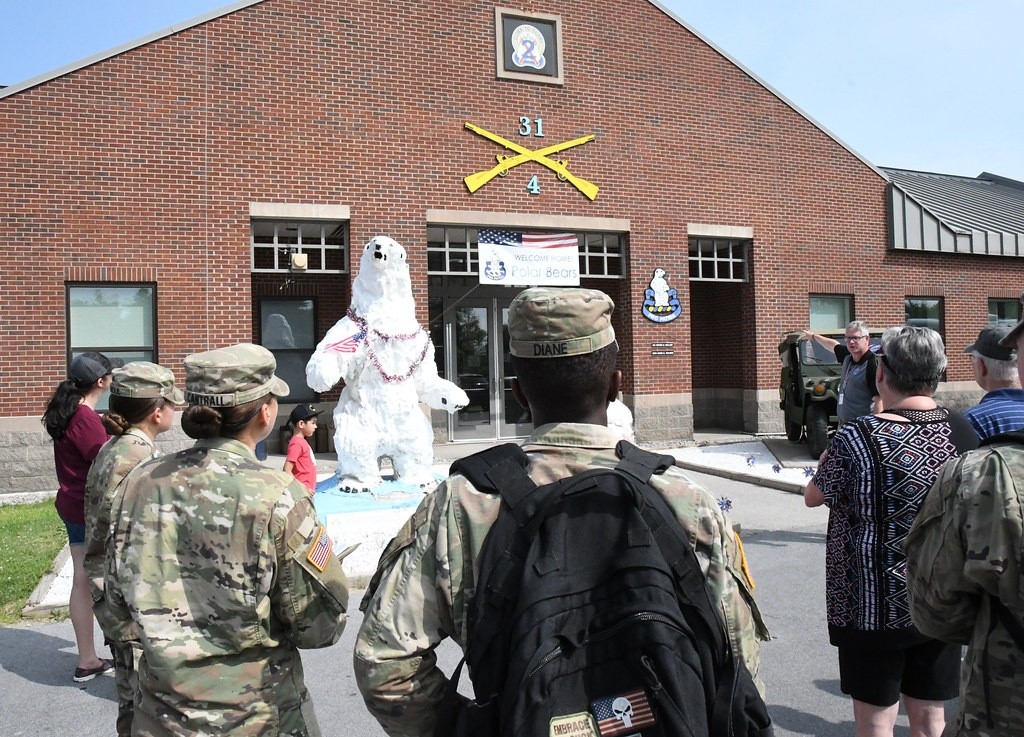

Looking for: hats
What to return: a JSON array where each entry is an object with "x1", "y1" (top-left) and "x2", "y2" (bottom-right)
[
  {"x1": 508, "y1": 287, "x2": 619, "y2": 356},
  {"x1": 998, "y1": 292, "x2": 1024, "y2": 348},
  {"x1": 69, "y1": 351, "x2": 124, "y2": 387},
  {"x1": 183, "y1": 343, "x2": 290, "y2": 408},
  {"x1": 110, "y1": 361, "x2": 185, "y2": 405},
  {"x1": 290, "y1": 404, "x2": 325, "y2": 423},
  {"x1": 961, "y1": 325, "x2": 1017, "y2": 361}
]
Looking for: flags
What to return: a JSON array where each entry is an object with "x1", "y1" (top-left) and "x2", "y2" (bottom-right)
[{"x1": 478, "y1": 229, "x2": 580, "y2": 287}]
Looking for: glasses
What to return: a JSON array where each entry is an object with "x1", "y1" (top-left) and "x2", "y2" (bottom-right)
[{"x1": 844, "y1": 336, "x2": 866, "y2": 342}]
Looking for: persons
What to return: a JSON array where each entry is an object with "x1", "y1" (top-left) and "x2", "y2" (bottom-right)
[
  {"x1": 40, "y1": 351, "x2": 116, "y2": 681},
  {"x1": 280, "y1": 402, "x2": 325, "y2": 503},
  {"x1": 606, "y1": 397, "x2": 633, "y2": 444},
  {"x1": 82, "y1": 361, "x2": 185, "y2": 737},
  {"x1": 901, "y1": 291, "x2": 1024, "y2": 737},
  {"x1": 103, "y1": 344, "x2": 349, "y2": 737},
  {"x1": 962, "y1": 326, "x2": 1024, "y2": 441},
  {"x1": 804, "y1": 327, "x2": 961, "y2": 737},
  {"x1": 353, "y1": 288, "x2": 774, "y2": 737},
  {"x1": 801, "y1": 321, "x2": 883, "y2": 433}
]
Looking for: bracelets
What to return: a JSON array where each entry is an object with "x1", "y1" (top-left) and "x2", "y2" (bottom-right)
[{"x1": 812, "y1": 333, "x2": 815, "y2": 340}]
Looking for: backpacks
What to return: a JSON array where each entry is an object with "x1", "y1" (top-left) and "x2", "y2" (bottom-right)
[{"x1": 448, "y1": 439, "x2": 776, "y2": 737}]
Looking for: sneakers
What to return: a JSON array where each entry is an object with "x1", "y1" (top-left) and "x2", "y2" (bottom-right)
[{"x1": 73, "y1": 658, "x2": 115, "y2": 683}]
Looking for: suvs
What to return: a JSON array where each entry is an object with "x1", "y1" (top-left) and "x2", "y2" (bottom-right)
[{"x1": 779, "y1": 328, "x2": 887, "y2": 460}]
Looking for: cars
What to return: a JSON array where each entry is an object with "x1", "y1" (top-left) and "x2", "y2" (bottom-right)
[{"x1": 458, "y1": 374, "x2": 489, "y2": 411}]
